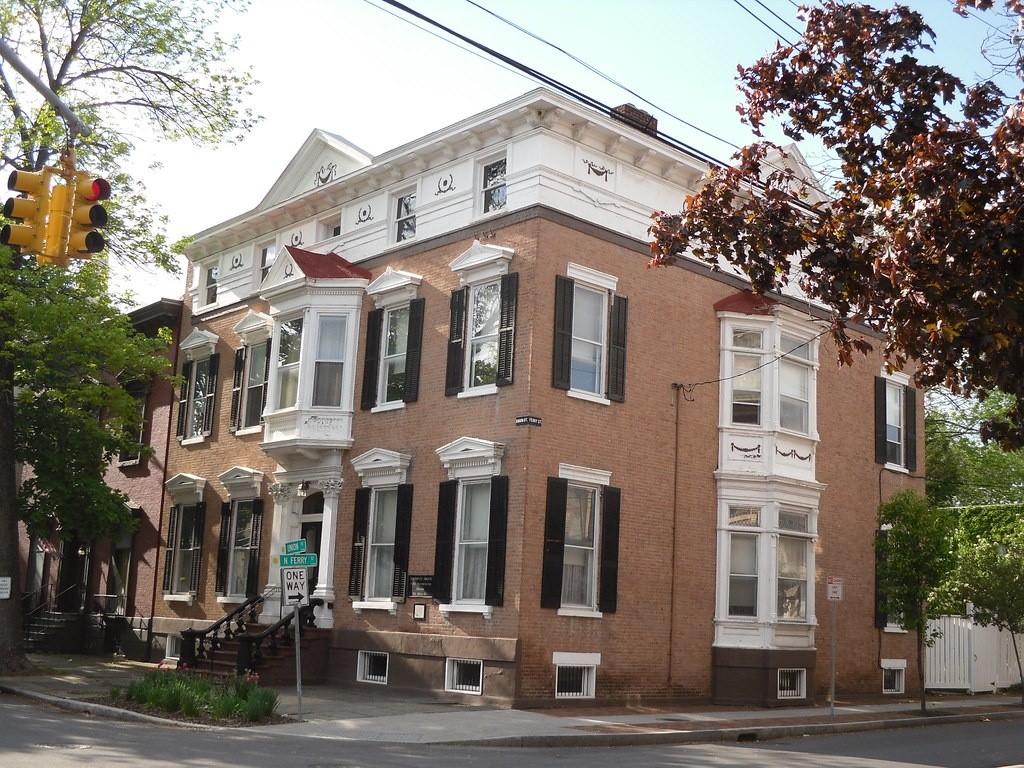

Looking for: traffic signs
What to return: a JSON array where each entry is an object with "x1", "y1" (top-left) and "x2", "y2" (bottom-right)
[
  {"x1": 281, "y1": 566, "x2": 310, "y2": 606},
  {"x1": 280, "y1": 538, "x2": 307, "y2": 555},
  {"x1": 270, "y1": 553, "x2": 318, "y2": 568}
]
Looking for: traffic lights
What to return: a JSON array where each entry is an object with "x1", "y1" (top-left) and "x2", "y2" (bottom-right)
[
  {"x1": 67, "y1": 171, "x2": 111, "y2": 260},
  {"x1": 0, "y1": 170, "x2": 41, "y2": 255}
]
